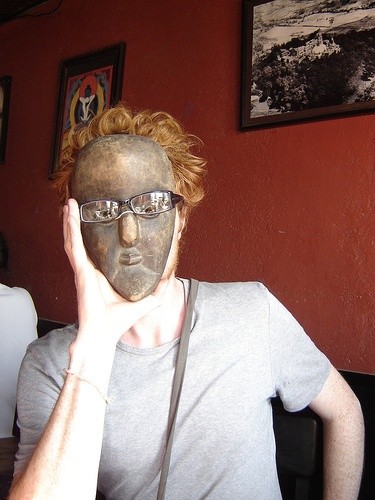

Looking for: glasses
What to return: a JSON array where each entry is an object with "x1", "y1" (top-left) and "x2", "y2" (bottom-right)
[{"x1": 79, "y1": 189, "x2": 182, "y2": 223}]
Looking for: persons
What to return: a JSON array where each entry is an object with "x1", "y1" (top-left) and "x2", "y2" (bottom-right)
[
  {"x1": 8, "y1": 107, "x2": 364, "y2": 500},
  {"x1": 0, "y1": 231, "x2": 39, "y2": 438}
]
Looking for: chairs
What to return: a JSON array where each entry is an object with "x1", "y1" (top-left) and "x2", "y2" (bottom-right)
[{"x1": 273, "y1": 411, "x2": 319, "y2": 500}]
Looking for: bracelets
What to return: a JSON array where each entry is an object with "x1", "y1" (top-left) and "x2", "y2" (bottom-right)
[{"x1": 64, "y1": 367, "x2": 110, "y2": 405}]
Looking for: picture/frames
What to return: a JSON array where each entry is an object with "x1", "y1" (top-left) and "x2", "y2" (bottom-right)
[
  {"x1": 238, "y1": 0, "x2": 375, "y2": 131},
  {"x1": 48, "y1": 41, "x2": 127, "y2": 179},
  {"x1": 0, "y1": 75, "x2": 11, "y2": 163}
]
